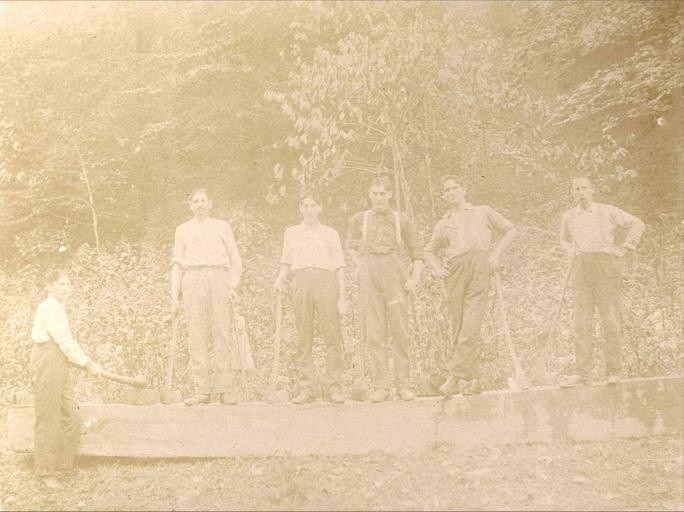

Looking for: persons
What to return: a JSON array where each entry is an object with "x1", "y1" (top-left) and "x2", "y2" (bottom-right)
[
  {"x1": 423, "y1": 173, "x2": 519, "y2": 397},
  {"x1": 556, "y1": 176, "x2": 647, "y2": 388},
  {"x1": 27, "y1": 271, "x2": 107, "y2": 491},
  {"x1": 273, "y1": 194, "x2": 350, "y2": 404},
  {"x1": 168, "y1": 188, "x2": 245, "y2": 405},
  {"x1": 346, "y1": 175, "x2": 427, "y2": 402}
]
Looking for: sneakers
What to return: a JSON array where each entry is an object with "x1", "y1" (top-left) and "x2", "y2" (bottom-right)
[
  {"x1": 438, "y1": 379, "x2": 460, "y2": 396},
  {"x1": 188, "y1": 394, "x2": 212, "y2": 407},
  {"x1": 373, "y1": 390, "x2": 389, "y2": 404},
  {"x1": 219, "y1": 391, "x2": 235, "y2": 405},
  {"x1": 460, "y1": 379, "x2": 480, "y2": 396},
  {"x1": 293, "y1": 391, "x2": 315, "y2": 404},
  {"x1": 43, "y1": 472, "x2": 60, "y2": 490},
  {"x1": 559, "y1": 374, "x2": 593, "y2": 389},
  {"x1": 397, "y1": 387, "x2": 412, "y2": 401},
  {"x1": 606, "y1": 375, "x2": 619, "y2": 386},
  {"x1": 326, "y1": 392, "x2": 344, "y2": 404}
]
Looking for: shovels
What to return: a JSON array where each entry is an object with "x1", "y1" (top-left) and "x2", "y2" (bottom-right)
[
  {"x1": 263, "y1": 289, "x2": 288, "y2": 407},
  {"x1": 494, "y1": 271, "x2": 531, "y2": 392}
]
